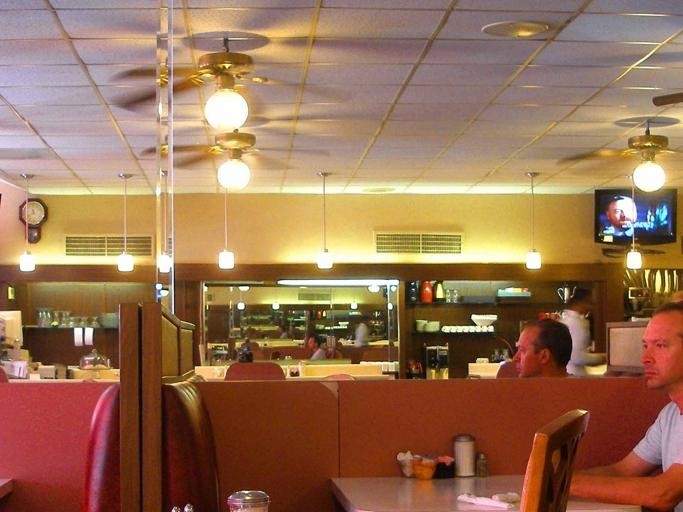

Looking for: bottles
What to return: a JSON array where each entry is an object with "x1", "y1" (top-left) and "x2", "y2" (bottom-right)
[
  {"x1": 226, "y1": 489, "x2": 271, "y2": 512},
  {"x1": 316, "y1": 309, "x2": 321, "y2": 320},
  {"x1": 446, "y1": 289, "x2": 458, "y2": 304},
  {"x1": 419, "y1": 281, "x2": 433, "y2": 304},
  {"x1": 476, "y1": 453, "x2": 488, "y2": 478},
  {"x1": 453, "y1": 434, "x2": 476, "y2": 477},
  {"x1": 13, "y1": 339, "x2": 21, "y2": 360}
]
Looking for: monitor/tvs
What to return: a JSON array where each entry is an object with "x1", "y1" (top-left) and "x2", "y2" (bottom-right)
[
  {"x1": 607, "y1": 322, "x2": 653, "y2": 375},
  {"x1": 594, "y1": 189, "x2": 677, "y2": 246}
]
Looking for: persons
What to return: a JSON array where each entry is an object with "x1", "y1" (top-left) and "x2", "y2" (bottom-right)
[
  {"x1": 353, "y1": 318, "x2": 369, "y2": 347},
  {"x1": 601, "y1": 195, "x2": 647, "y2": 237},
  {"x1": 512, "y1": 318, "x2": 576, "y2": 378},
  {"x1": 306, "y1": 335, "x2": 327, "y2": 361},
  {"x1": 572, "y1": 301, "x2": 683, "y2": 512}
]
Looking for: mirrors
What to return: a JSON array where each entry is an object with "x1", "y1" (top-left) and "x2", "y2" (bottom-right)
[{"x1": 202, "y1": 285, "x2": 390, "y2": 366}]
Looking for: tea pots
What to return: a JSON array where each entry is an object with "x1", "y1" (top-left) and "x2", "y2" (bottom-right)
[
  {"x1": 322, "y1": 310, "x2": 327, "y2": 317},
  {"x1": 556, "y1": 284, "x2": 577, "y2": 304},
  {"x1": 372, "y1": 311, "x2": 381, "y2": 318},
  {"x1": 408, "y1": 359, "x2": 422, "y2": 380},
  {"x1": 433, "y1": 280, "x2": 446, "y2": 304}
]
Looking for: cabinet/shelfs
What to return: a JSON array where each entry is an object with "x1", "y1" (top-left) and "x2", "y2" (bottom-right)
[{"x1": 407, "y1": 300, "x2": 529, "y2": 335}]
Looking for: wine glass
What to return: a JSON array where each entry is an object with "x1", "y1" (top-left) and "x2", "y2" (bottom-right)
[
  {"x1": 90, "y1": 316, "x2": 99, "y2": 327},
  {"x1": 36, "y1": 306, "x2": 88, "y2": 327}
]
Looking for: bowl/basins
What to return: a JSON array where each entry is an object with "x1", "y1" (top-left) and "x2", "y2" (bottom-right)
[
  {"x1": 409, "y1": 460, "x2": 438, "y2": 480},
  {"x1": 339, "y1": 322, "x2": 350, "y2": 326},
  {"x1": 470, "y1": 314, "x2": 498, "y2": 326}
]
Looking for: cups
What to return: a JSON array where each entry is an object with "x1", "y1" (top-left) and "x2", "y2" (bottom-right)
[
  {"x1": 285, "y1": 356, "x2": 292, "y2": 360},
  {"x1": 289, "y1": 367, "x2": 300, "y2": 377},
  {"x1": 333, "y1": 325, "x2": 347, "y2": 329},
  {"x1": 441, "y1": 326, "x2": 494, "y2": 333}
]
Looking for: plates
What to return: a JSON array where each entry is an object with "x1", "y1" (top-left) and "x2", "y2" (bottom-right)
[
  {"x1": 316, "y1": 324, "x2": 324, "y2": 329},
  {"x1": 415, "y1": 319, "x2": 440, "y2": 333}
]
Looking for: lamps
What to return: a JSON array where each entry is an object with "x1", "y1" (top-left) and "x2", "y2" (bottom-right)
[
  {"x1": 198, "y1": 39, "x2": 253, "y2": 132},
  {"x1": 524, "y1": 171, "x2": 542, "y2": 271},
  {"x1": 117, "y1": 173, "x2": 134, "y2": 272},
  {"x1": 219, "y1": 188, "x2": 235, "y2": 270},
  {"x1": 215, "y1": 132, "x2": 256, "y2": 192},
  {"x1": 318, "y1": 171, "x2": 333, "y2": 268},
  {"x1": 628, "y1": 121, "x2": 669, "y2": 191},
  {"x1": 19, "y1": 173, "x2": 37, "y2": 272}
]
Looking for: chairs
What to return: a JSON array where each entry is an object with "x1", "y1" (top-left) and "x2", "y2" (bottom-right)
[
  {"x1": 141, "y1": 302, "x2": 220, "y2": 512},
  {"x1": 497, "y1": 362, "x2": 519, "y2": 377},
  {"x1": 224, "y1": 363, "x2": 286, "y2": 380},
  {"x1": 83, "y1": 304, "x2": 141, "y2": 511},
  {"x1": 520, "y1": 409, "x2": 591, "y2": 511}
]
